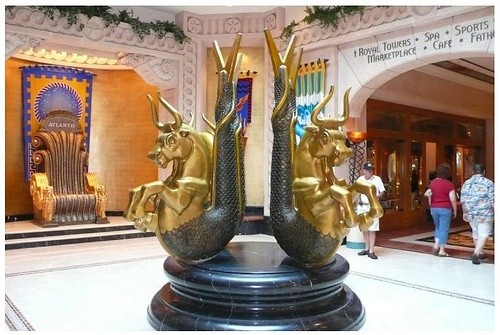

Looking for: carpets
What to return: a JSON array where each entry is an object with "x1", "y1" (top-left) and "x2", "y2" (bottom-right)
[{"x1": 417, "y1": 227, "x2": 493, "y2": 250}]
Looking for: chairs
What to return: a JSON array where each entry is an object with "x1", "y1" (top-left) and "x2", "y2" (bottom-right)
[{"x1": 29, "y1": 130, "x2": 110, "y2": 228}]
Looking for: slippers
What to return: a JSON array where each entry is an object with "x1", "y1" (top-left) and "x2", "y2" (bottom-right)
[
  {"x1": 437, "y1": 251, "x2": 450, "y2": 257},
  {"x1": 433, "y1": 248, "x2": 439, "y2": 252}
]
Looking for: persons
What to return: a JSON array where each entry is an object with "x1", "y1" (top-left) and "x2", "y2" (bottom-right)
[
  {"x1": 459, "y1": 163, "x2": 495, "y2": 264},
  {"x1": 410, "y1": 169, "x2": 419, "y2": 211},
  {"x1": 356, "y1": 162, "x2": 386, "y2": 259},
  {"x1": 424, "y1": 165, "x2": 457, "y2": 257}
]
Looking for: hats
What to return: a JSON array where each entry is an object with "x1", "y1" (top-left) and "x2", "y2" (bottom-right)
[{"x1": 364, "y1": 163, "x2": 373, "y2": 169}]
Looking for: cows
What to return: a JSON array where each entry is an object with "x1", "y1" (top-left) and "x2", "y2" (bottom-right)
[
  {"x1": 120, "y1": 88, "x2": 216, "y2": 237},
  {"x1": 290, "y1": 83, "x2": 385, "y2": 240}
]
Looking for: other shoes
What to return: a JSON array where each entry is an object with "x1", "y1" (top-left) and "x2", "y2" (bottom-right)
[
  {"x1": 479, "y1": 253, "x2": 486, "y2": 260},
  {"x1": 472, "y1": 254, "x2": 480, "y2": 264},
  {"x1": 368, "y1": 252, "x2": 378, "y2": 259},
  {"x1": 358, "y1": 250, "x2": 369, "y2": 255}
]
